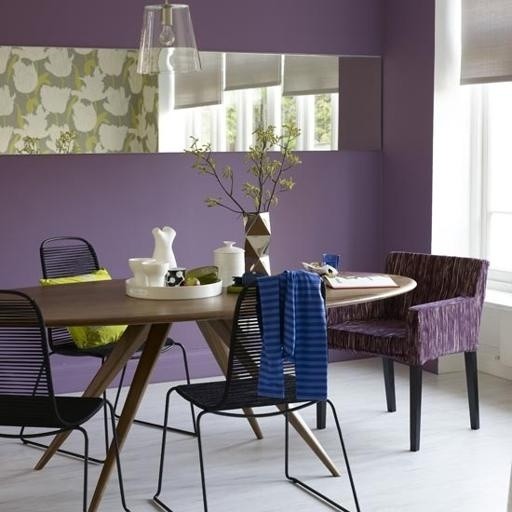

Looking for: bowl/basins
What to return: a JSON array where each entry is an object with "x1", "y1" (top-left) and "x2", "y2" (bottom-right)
[{"x1": 187, "y1": 266, "x2": 219, "y2": 284}]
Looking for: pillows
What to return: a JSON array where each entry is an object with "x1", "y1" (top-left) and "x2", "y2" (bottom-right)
[{"x1": 38, "y1": 267, "x2": 129, "y2": 349}]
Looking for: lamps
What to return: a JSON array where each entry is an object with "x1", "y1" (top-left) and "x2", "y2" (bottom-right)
[{"x1": 136, "y1": 0, "x2": 202, "y2": 73}]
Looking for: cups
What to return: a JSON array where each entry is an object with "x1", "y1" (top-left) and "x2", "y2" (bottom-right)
[
  {"x1": 142, "y1": 260, "x2": 169, "y2": 286},
  {"x1": 213, "y1": 240, "x2": 246, "y2": 287},
  {"x1": 165, "y1": 269, "x2": 186, "y2": 287},
  {"x1": 128, "y1": 257, "x2": 156, "y2": 287}
]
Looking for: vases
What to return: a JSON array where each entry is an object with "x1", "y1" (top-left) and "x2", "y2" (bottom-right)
[{"x1": 244, "y1": 212, "x2": 271, "y2": 282}]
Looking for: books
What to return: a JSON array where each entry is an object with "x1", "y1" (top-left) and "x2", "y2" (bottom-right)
[{"x1": 322, "y1": 274, "x2": 400, "y2": 290}]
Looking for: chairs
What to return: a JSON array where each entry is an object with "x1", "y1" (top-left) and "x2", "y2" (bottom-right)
[
  {"x1": 316, "y1": 252, "x2": 489, "y2": 452},
  {"x1": 153, "y1": 274, "x2": 360, "y2": 512},
  {"x1": 0, "y1": 289, "x2": 129, "y2": 512},
  {"x1": 19, "y1": 235, "x2": 199, "y2": 464}
]
